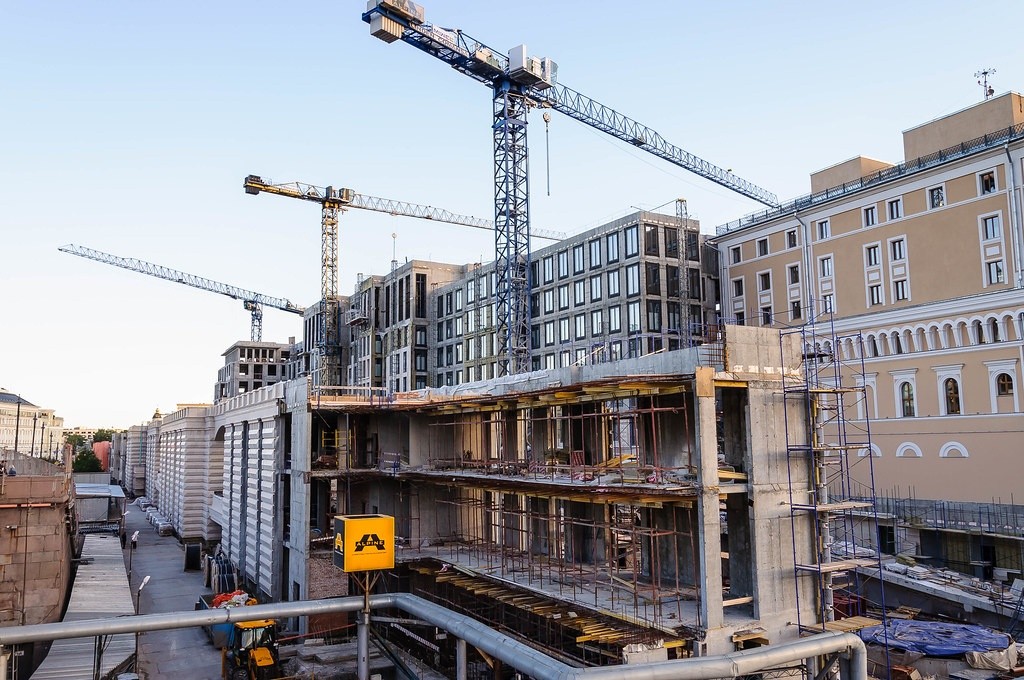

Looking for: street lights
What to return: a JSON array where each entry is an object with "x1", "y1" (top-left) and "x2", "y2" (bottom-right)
[
  {"x1": 40, "y1": 421, "x2": 45, "y2": 458},
  {"x1": 134, "y1": 574, "x2": 152, "y2": 673},
  {"x1": 49, "y1": 431, "x2": 54, "y2": 462},
  {"x1": 122, "y1": 510, "x2": 130, "y2": 534},
  {"x1": 128, "y1": 530, "x2": 141, "y2": 586},
  {"x1": 30, "y1": 412, "x2": 38, "y2": 456},
  {"x1": 13, "y1": 392, "x2": 24, "y2": 452}
]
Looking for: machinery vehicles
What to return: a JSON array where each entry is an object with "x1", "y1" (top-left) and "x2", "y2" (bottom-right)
[{"x1": 222, "y1": 598, "x2": 284, "y2": 680}]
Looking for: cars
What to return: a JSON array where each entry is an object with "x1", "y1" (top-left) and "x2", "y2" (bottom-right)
[{"x1": 136, "y1": 496, "x2": 173, "y2": 537}]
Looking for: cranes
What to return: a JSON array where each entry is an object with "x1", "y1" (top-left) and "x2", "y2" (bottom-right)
[
  {"x1": 362, "y1": 0, "x2": 779, "y2": 381},
  {"x1": 57, "y1": 243, "x2": 307, "y2": 342},
  {"x1": 242, "y1": 173, "x2": 570, "y2": 396}
]
